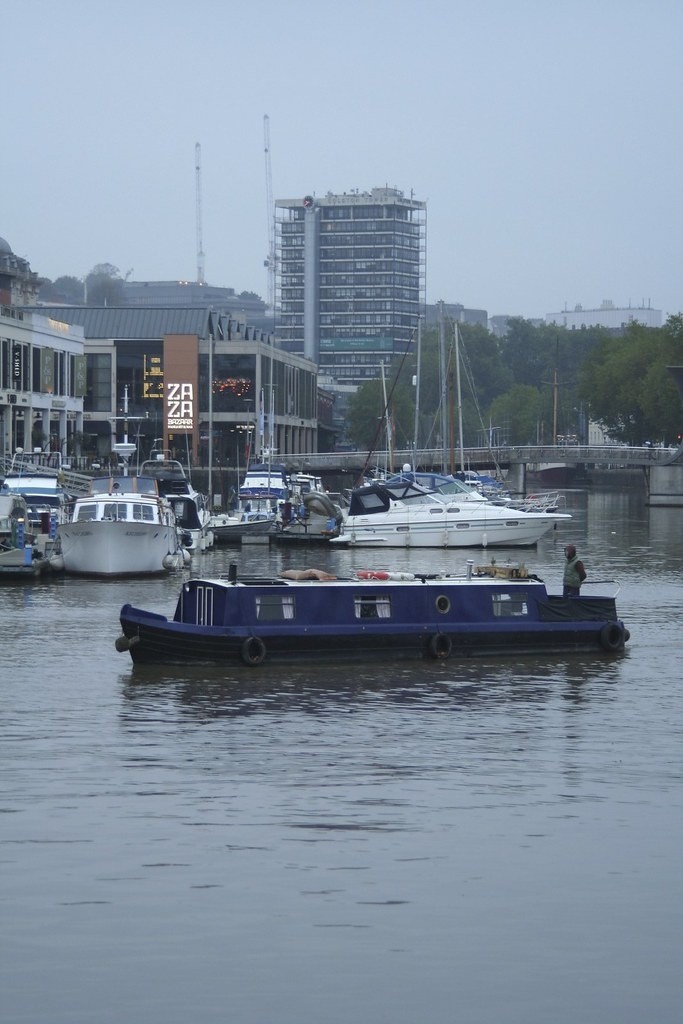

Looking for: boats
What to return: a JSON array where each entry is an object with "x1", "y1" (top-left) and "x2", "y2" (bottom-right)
[
  {"x1": 226, "y1": 316, "x2": 581, "y2": 547},
  {"x1": 0, "y1": 384, "x2": 274, "y2": 577},
  {"x1": 116, "y1": 557, "x2": 630, "y2": 665}
]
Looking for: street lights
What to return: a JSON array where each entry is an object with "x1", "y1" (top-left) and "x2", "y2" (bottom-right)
[{"x1": 243, "y1": 399, "x2": 253, "y2": 465}]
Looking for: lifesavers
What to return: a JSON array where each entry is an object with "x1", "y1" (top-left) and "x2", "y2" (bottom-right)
[
  {"x1": 241, "y1": 636, "x2": 267, "y2": 667},
  {"x1": 429, "y1": 632, "x2": 453, "y2": 659},
  {"x1": 356, "y1": 570, "x2": 417, "y2": 582},
  {"x1": 600, "y1": 622, "x2": 623, "y2": 650}
]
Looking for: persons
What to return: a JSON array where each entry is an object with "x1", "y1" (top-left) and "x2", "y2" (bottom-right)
[
  {"x1": 95, "y1": 456, "x2": 118, "y2": 469},
  {"x1": 563, "y1": 544, "x2": 586, "y2": 597}
]
